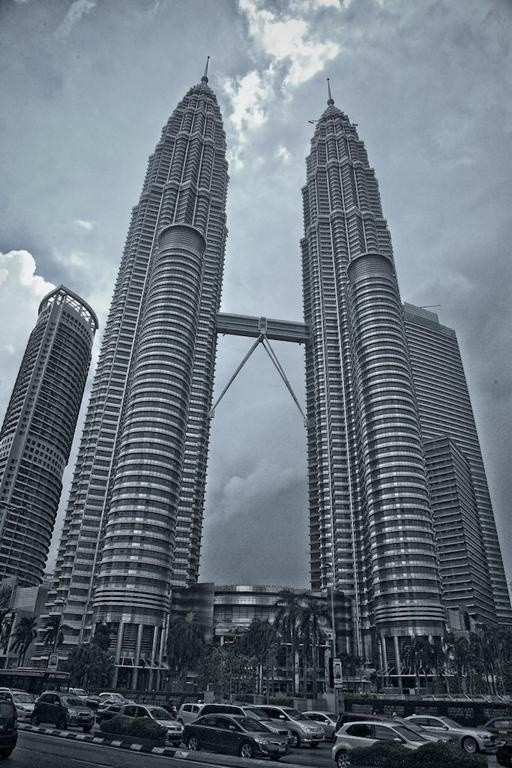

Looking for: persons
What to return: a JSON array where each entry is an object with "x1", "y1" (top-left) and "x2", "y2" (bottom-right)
[{"x1": 171, "y1": 704, "x2": 177, "y2": 712}]
